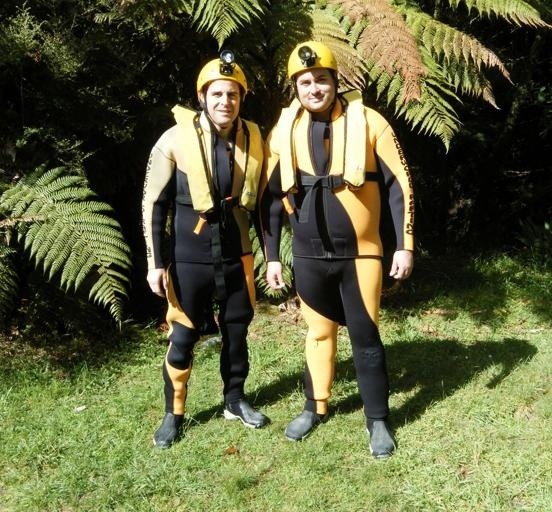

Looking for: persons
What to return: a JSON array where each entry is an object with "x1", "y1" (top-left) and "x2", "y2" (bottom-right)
[
  {"x1": 141, "y1": 48, "x2": 268, "y2": 450},
  {"x1": 256, "y1": 39, "x2": 417, "y2": 459}
]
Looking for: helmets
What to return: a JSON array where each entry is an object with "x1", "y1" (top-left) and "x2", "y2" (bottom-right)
[
  {"x1": 196, "y1": 57, "x2": 248, "y2": 104},
  {"x1": 287, "y1": 40, "x2": 337, "y2": 80}
]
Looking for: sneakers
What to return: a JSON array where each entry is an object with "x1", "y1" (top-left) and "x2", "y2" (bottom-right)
[
  {"x1": 151, "y1": 411, "x2": 184, "y2": 449},
  {"x1": 365, "y1": 418, "x2": 398, "y2": 460},
  {"x1": 284, "y1": 410, "x2": 321, "y2": 443},
  {"x1": 222, "y1": 401, "x2": 270, "y2": 428}
]
